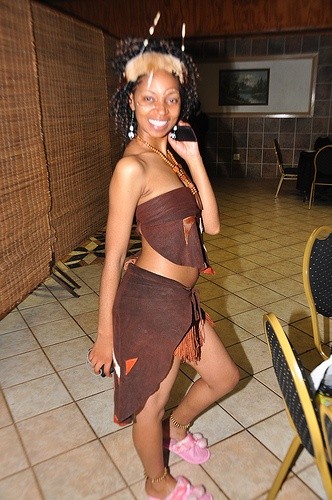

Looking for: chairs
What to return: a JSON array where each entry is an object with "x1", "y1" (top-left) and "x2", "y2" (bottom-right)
[
  {"x1": 263, "y1": 312, "x2": 332, "y2": 500},
  {"x1": 302, "y1": 224, "x2": 332, "y2": 360},
  {"x1": 273, "y1": 136, "x2": 332, "y2": 210}
]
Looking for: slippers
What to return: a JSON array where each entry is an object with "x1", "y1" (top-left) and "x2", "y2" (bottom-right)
[
  {"x1": 163, "y1": 430, "x2": 211, "y2": 465},
  {"x1": 150, "y1": 475, "x2": 214, "y2": 500}
]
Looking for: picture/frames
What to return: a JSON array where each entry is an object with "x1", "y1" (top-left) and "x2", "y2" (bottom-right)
[{"x1": 197, "y1": 50, "x2": 320, "y2": 118}]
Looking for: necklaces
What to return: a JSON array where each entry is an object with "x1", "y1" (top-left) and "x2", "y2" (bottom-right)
[{"x1": 134, "y1": 133, "x2": 204, "y2": 211}]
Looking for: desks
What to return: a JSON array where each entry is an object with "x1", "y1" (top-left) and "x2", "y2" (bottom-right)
[{"x1": 296, "y1": 150, "x2": 317, "y2": 202}]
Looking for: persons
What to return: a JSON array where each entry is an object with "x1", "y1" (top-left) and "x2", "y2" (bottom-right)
[{"x1": 88, "y1": 11, "x2": 239, "y2": 500}]
